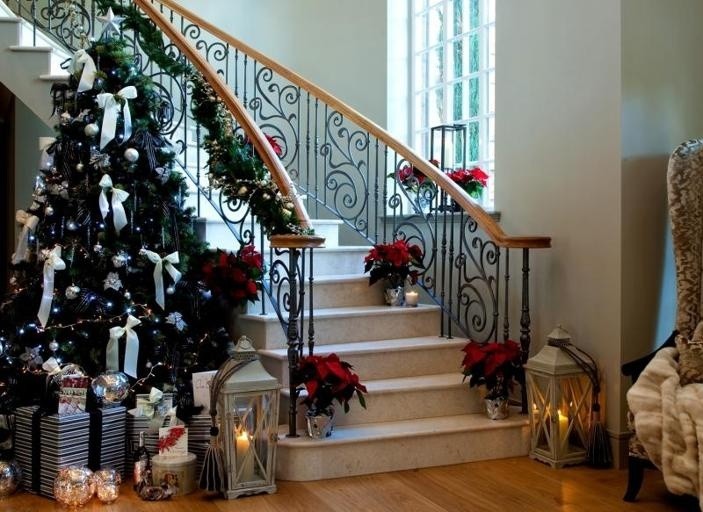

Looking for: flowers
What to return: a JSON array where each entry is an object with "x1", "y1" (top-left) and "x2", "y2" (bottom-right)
[
  {"x1": 235, "y1": 130, "x2": 285, "y2": 165},
  {"x1": 360, "y1": 240, "x2": 428, "y2": 290},
  {"x1": 442, "y1": 162, "x2": 490, "y2": 202},
  {"x1": 291, "y1": 347, "x2": 369, "y2": 416},
  {"x1": 384, "y1": 155, "x2": 441, "y2": 195},
  {"x1": 197, "y1": 241, "x2": 268, "y2": 324},
  {"x1": 458, "y1": 336, "x2": 526, "y2": 401}
]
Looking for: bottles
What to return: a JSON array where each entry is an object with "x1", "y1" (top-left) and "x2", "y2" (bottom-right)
[{"x1": 132, "y1": 430, "x2": 150, "y2": 492}]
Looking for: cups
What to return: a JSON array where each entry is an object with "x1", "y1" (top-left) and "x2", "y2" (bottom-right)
[{"x1": 405, "y1": 292, "x2": 417, "y2": 307}]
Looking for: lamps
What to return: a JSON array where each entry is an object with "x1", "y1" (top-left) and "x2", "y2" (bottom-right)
[
  {"x1": 197, "y1": 335, "x2": 284, "y2": 503},
  {"x1": 521, "y1": 321, "x2": 616, "y2": 475}
]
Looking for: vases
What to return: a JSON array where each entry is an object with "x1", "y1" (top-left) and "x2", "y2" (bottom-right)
[
  {"x1": 383, "y1": 284, "x2": 405, "y2": 307},
  {"x1": 305, "y1": 402, "x2": 334, "y2": 440},
  {"x1": 484, "y1": 391, "x2": 510, "y2": 421}
]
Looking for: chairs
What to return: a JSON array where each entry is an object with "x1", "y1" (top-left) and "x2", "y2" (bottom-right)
[{"x1": 621, "y1": 137, "x2": 703, "y2": 512}]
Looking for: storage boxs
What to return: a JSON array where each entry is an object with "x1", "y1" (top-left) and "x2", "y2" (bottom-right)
[
  {"x1": 15, "y1": 403, "x2": 129, "y2": 501},
  {"x1": 129, "y1": 410, "x2": 212, "y2": 481}
]
